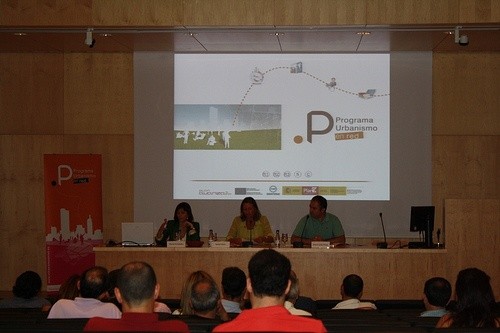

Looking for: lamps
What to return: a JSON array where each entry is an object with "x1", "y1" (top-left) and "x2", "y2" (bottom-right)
[
  {"x1": 84, "y1": 28, "x2": 92, "y2": 45},
  {"x1": 455, "y1": 25, "x2": 469, "y2": 46}
]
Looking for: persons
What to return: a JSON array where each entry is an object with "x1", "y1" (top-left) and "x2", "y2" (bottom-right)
[
  {"x1": 41, "y1": 266, "x2": 124, "y2": 319},
  {"x1": 290, "y1": 196, "x2": 345, "y2": 247},
  {"x1": 0, "y1": 270, "x2": 50, "y2": 308},
  {"x1": 212, "y1": 248, "x2": 327, "y2": 333},
  {"x1": 420, "y1": 277, "x2": 452, "y2": 317},
  {"x1": 155, "y1": 202, "x2": 198, "y2": 244},
  {"x1": 435, "y1": 268, "x2": 500, "y2": 329},
  {"x1": 154, "y1": 302, "x2": 172, "y2": 313},
  {"x1": 331, "y1": 274, "x2": 377, "y2": 309},
  {"x1": 99, "y1": 269, "x2": 122, "y2": 312},
  {"x1": 44, "y1": 274, "x2": 81, "y2": 306},
  {"x1": 172, "y1": 270, "x2": 228, "y2": 320},
  {"x1": 221, "y1": 267, "x2": 247, "y2": 320},
  {"x1": 82, "y1": 262, "x2": 188, "y2": 333},
  {"x1": 226, "y1": 197, "x2": 273, "y2": 245},
  {"x1": 284, "y1": 270, "x2": 312, "y2": 316},
  {"x1": 181, "y1": 281, "x2": 226, "y2": 333}
]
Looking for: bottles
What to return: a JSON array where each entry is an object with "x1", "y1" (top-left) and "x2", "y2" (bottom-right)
[
  {"x1": 274, "y1": 230, "x2": 280, "y2": 248},
  {"x1": 208, "y1": 229, "x2": 214, "y2": 247}
]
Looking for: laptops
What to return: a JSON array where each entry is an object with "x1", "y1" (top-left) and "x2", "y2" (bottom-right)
[{"x1": 122, "y1": 222, "x2": 153, "y2": 246}]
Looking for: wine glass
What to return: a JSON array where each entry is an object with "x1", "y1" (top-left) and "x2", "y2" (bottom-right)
[{"x1": 281, "y1": 233, "x2": 288, "y2": 248}]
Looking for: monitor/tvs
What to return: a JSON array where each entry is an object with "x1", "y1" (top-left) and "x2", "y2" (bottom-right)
[{"x1": 408, "y1": 206, "x2": 435, "y2": 249}]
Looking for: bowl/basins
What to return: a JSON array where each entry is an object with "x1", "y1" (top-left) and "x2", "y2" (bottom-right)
[{"x1": 187, "y1": 240, "x2": 204, "y2": 247}]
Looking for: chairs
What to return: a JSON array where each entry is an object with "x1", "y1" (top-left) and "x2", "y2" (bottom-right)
[{"x1": 0, "y1": 296, "x2": 500, "y2": 333}]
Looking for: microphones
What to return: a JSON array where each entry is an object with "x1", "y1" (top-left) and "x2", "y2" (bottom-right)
[
  {"x1": 242, "y1": 220, "x2": 253, "y2": 247},
  {"x1": 292, "y1": 214, "x2": 310, "y2": 248},
  {"x1": 377, "y1": 213, "x2": 388, "y2": 249}
]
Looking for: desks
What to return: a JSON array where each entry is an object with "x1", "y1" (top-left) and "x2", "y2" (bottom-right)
[{"x1": 93, "y1": 247, "x2": 448, "y2": 301}]
